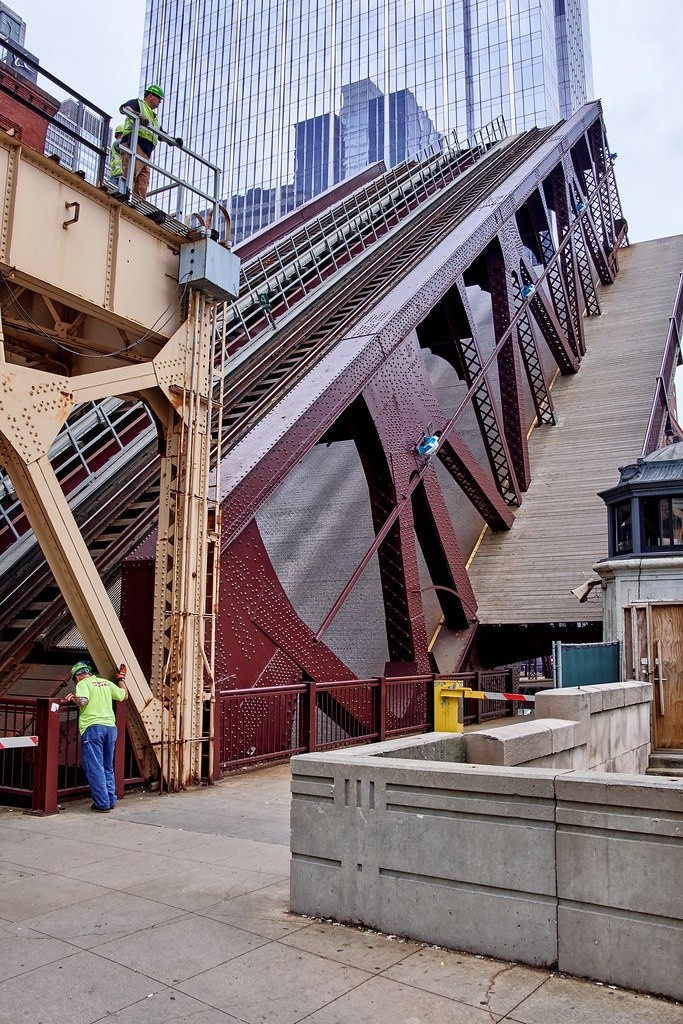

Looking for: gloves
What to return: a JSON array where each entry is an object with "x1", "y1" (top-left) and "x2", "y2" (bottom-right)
[
  {"x1": 169, "y1": 137, "x2": 183, "y2": 148},
  {"x1": 129, "y1": 111, "x2": 150, "y2": 126},
  {"x1": 115, "y1": 663, "x2": 127, "y2": 682},
  {"x1": 64, "y1": 692, "x2": 75, "y2": 701}
]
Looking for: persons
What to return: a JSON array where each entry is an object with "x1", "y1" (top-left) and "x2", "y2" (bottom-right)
[
  {"x1": 118, "y1": 84, "x2": 183, "y2": 203},
  {"x1": 63, "y1": 662, "x2": 129, "y2": 813},
  {"x1": 110, "y1": 123, "x2": 125, "y2": 185}
]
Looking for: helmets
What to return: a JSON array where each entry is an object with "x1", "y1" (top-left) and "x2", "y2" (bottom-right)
[
  {"x1": 69, "y1": 661, "x2": 92, "y2": 683},
  {"x1": 115, "y1": 125, "x2": 124, "y2": 133},
  {"x1": 144, "y1": 85, "x2": 164, "y2": 99}
]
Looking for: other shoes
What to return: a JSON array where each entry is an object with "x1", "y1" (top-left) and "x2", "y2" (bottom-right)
[{"x1": 91, "y1": 803, "x2": 110, "y2": 812}]
